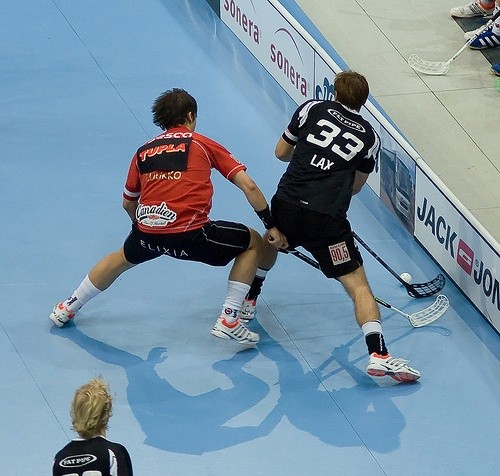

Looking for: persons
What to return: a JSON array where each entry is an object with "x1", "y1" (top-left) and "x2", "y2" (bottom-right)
[
  {"x1": 51, "y1": 376, "x2": 133, "y2": 476},
  {"x1": 240, "y1": 70, "x2": 421, "y2": 382},
  {"x1": 49, "y1": 88, "x2": 289, "y2": 345}
]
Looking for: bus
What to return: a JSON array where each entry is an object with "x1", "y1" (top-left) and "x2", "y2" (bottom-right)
[{"x1": 382, "y1": 146, "x2": 415, "y2": 222}]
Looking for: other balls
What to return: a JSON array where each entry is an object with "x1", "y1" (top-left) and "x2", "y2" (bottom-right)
[{"x1": 399, "y1": 272, "x2": 413, "y2": 284}]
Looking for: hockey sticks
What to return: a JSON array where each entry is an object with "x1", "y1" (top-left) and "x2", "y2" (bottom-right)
[
  {"x1": 351, "y1": 226, "x2": 446, "y2": 299},
  {"x1": 408, "y1": 9, "x2": 500, "y2": 76},
  {"x1": 269, "y1": 237, "x2": 449, "y2": 328}
]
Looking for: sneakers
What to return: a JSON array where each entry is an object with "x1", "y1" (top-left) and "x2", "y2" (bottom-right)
[
  {"x1": 450, "y1": 0, "x2": 496, "y2": 18},
  {"x1": 464, "y1": 20, "x2": 493, "y2": 40},
  {"x1": 491, "y1": 64, "x2": 500, "y2": 75},
  {"x1": 50, "y1": 301, "x2": 75, "y2": 328},
  {"x1": 367, "y1": 352, "x2": 421, "y2": 381},
  {"x1": 211, "y1": 313, "x2": 260, "y2": 348},
  {"x1": 239, "y1": 298, "x2": 257, "y2": 319},
  {"x1": 467, "y1": 26, "x2": 500, "y2": 49}
]
